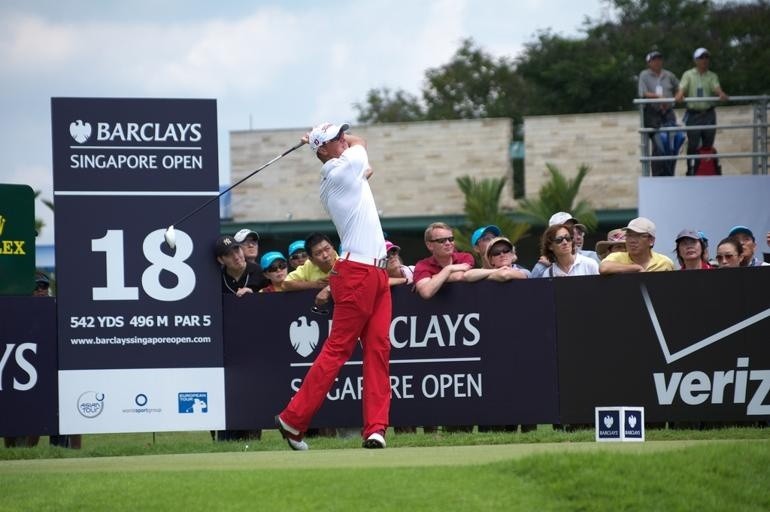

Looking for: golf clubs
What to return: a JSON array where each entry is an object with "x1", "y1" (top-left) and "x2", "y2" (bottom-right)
[{"x1": 165, "y1": 142, "x2": 305, "y2": 248}]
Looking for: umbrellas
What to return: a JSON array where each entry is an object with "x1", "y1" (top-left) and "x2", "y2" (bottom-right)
[{"x1": 653, "y1": 106, "x2": 687, "y2": 175}]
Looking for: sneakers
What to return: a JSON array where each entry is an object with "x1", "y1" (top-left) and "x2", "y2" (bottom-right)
[
  {"x1": 274, "y1": 414, "x2": 309, "y2": 450},
  {"x1": 364, "y1": 432, "x2": 387, "y2": 448}
]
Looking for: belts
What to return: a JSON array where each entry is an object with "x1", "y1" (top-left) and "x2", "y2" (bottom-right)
[{"x1": 338, "y1": 250, "x2": 390, "y2": 270}]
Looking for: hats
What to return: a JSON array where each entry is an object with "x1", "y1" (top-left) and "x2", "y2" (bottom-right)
[
  {"x1": 727, "y1": 225, "x2": 754, "y2": 239},
  {"x1": 644, "y1": 50, "x2": 663, "y2": 63},
  {"x1": 214, "y1": 233, "x2": 242, "y2": 258},
  {"x1": 259, "y1": 250, "x2": 289, "y2": 271},
  {"x1": 548, "y1": 211, "x2": 578, "y2": 229},
  {"x1": 567, "y1": 223, "x2": 590, "y2": 234},
  {"x1": 470, "y1": 224, "x2": 502, "y2": 249},
  {"x1": 233, "y1": 228, "x2": 260, "y2": 242},
  {"x1": 484, "y1": 236, "x2": 515, "y2": 263},
  {"x1": 594, "y1": 227, "x2": 627, "y2": 262},
  {"x1": 308, "y1": 122, "x2": 350, "y2": 155},
  {"x1": 385, "y1": 240, "x2": 401, "y2": 253},
  {"x1": 622, "y1": 216, "x2": 657, "y2": 239},
  {"x1": 34, "y1": 271, "x2": 50, "y2": 284},
  {"x1": 697, "y1": 230, "x2": 710, "y2": 242},
  {"x1": 674, "y1": 227, "x2": 700, "y2": 243},
  {"x1": 691, "y1": 47, "x2": 713, "y2": 62},
  {"x1": 287, "y1": 240, "x2": 307, "y2": 257}
]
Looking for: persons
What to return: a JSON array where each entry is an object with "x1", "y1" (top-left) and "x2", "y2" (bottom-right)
[
  {"x1": 274, "y1": 123, "x2": 392, "y2": 450},
  {"x1": 675, "y1": 48, "x2": 726, "y2": 176},
  {"x1": 31, "y1": 271, "x2": 50, "y2": 296},
  {"x1": 637, "y1": 51, "x2": 684, "y2": 176}
]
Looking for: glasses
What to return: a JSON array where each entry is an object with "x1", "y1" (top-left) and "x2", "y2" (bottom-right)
[
  {"x1": 268, "y1": 263, "x2": 288, "y2": 272},
  {"x1": 714, "y1": 252, "x2": 739, "y2": 263},
  {"x1": 550, "y1": 236, "x2": 571, "y2": 244},
  {"x1": 329, "y1": 130, "x2": 345, "y2": 144},
  {"x1": 428, "y1": 236, "x2": 455, "y2": 244},
  {"x1": 34, "y1": 281, "x2": 50, "y2": 291},
  {"x1": 490, "y1": 245, "x2": 512, "y2": 258}
]
